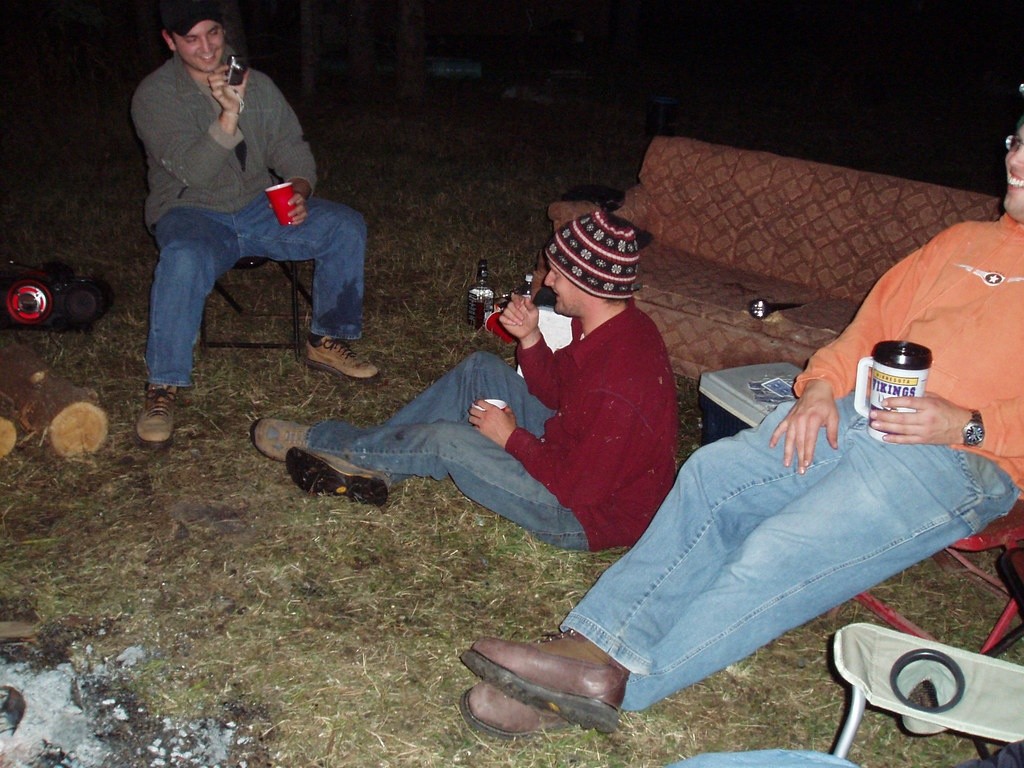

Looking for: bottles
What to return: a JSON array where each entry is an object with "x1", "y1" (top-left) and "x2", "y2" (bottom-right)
[
  {"x1": 520, "y1": 273, "x2": 534, "y2": 299},
  {"x1": 467, "y1": 259, "x2": 496, "y2": 329}
]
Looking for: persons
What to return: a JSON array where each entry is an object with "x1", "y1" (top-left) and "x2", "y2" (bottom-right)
[
  {"x1": 130, "y1": 1, "x2": 381, "y2": 448},
  {"x1": 253, "y1": 210, "x2": 677, "y2": 551},
  {"x1": 459, "y1": 123, "x2": 1024, "y2": 739},
  {"x1": 666, "y1": 739, "x2": 1024, "y2": 767}
]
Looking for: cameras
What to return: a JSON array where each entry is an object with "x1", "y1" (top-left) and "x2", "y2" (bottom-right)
[{"x1": 226, "y1": 54, "x2": 247, "y2": 86}]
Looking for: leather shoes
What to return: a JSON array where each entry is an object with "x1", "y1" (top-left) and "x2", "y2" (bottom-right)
[
  {"x1": 458, "y1": 629, "x2": 630, "y2": 734},
  {"x1": 460, "y1": 681, "x2": 571, "y2": 740}
]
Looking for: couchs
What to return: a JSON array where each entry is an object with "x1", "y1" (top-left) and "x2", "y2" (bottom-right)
[{"x1": 529, "y1": 134, "x2": 1006, "y2": 385}]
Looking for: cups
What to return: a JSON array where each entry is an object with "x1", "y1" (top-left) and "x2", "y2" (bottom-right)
[
  {"x1": 853, "y1": 340, "x2": 932, "y2": 440},
  {"x1": 471, "y1": 398, "x2": 507, "y2": 429},
  {"x1": 484, "y1": 311, "x2": 517, "y2": 344},
  {"x1": 264, "y1": 182, "x2": 300, "y2": 227}
]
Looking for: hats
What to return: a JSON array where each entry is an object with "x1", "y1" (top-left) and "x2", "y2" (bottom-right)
[
  {"x1": 544, "y1": 211, "x2": 640, "y2": 299},
  {"x1": 159, "y1": 0, "x2": 224, "y2": 36}
]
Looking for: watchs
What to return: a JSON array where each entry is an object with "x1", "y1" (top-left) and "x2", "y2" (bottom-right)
[{"x1": 962, "y1": 409, "x2": 985, "y2": 446}]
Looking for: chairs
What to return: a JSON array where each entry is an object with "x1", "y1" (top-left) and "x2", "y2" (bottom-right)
[
  {"x1": 828, "y1": 499, "x2": 1024, "y2": 657},
  {"x1": 661, "y1": 621, "x2": 1024, "y2": 768},
  {"x1": 200, "y1": 256, "x2": 314, "y2": 363}
]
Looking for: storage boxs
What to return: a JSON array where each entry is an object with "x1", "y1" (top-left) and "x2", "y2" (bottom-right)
[{"x1": 697, "y1": 361, "x2": 804, "y2": 448}]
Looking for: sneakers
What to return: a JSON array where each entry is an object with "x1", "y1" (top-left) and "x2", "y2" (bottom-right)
[
  {"x1": 285, "y1": 445, "x2": 391, "y2": 507},
  {"x1": 135, "y1": 383, "x2": 178, "y2": 444},
  {"x1": 249, "y1": 418, "x2": 313, "y2": 463},
  {"x1": 303, "y1": 335, "x2": 381, "y2": 381}
]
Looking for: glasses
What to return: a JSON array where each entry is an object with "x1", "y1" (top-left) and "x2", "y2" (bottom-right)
[{"x1": 1005, "y1": 135, "x2": 1024, "y2": 153}]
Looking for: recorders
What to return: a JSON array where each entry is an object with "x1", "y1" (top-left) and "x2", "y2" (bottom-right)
[{"x1": 0, "y1": 259, "x2": 115, "y2": 333}]
